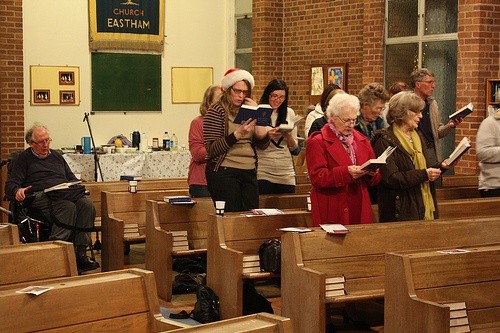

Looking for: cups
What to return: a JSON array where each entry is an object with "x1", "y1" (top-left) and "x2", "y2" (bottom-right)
[
  {"x1": 215, "y1": 201, "x2": 225, "y2": 214},
  {"x1": 129, "y1": 181, "x2": 137, "y2": 192},
  {"x1": 103, "y1": 145, "x2": 115, "y2": 154},
  {"x1": 76, "y1": 144, "x2": 82, "y2": 152},
  {"x1": 81, "y1": 137, "x2": 90, "y2": 153},
  {"x1": 306, "y1": 197, "x2": 312, "y2": 210}
]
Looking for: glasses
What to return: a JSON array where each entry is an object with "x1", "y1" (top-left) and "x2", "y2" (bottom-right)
[
  {"x1": 419, "y1": 81, "x2": 436, "y2": 85},
  {"x1": 409, "y1": 110, "x2": 423, "y2": 115},
  {"x1": 374, "y1": 106, "x2": 386, "y2": 111},
  {"x1": 231, "y1": 88, "x2": 249, "y2": 95},
  {"x1": 271, "y1": 94, "x2": 286, "y2": 100},
  {"x1": 338, "y1": 116, "x2": 360, "y2": 126},
  {"x1": 32, "y1": 137, "x2": 52, "y2": 145}
]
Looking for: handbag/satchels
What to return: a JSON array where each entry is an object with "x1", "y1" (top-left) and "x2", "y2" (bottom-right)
[
  {"x1": 193, "y1": 284, "x2": 220, "y2": 324},
  {"x1": 172, "y1": 271, "x2": 207, "y2": 294},
  {"x1": 45, "y1": 185, "x2": 86, "y2": 201},
  {"x1": 173, "y1": 254, "x2": 207, "y2": 273},
  {"x1": 259, "y1": 238, "x2": 281, "y2": 272}
]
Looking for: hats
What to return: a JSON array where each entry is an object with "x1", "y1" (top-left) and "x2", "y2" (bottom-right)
[{"x1": 222, "y1": 68, "x2": 255, "y2": 91}]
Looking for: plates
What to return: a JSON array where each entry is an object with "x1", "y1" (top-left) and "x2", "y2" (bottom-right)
[{"x1": 97, "y1": 151, "x2": 105, "y2": 154}]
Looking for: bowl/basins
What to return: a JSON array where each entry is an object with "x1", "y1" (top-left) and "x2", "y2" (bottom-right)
[
  {"x1": 127, "y1": 148, "x2": 137, "y2": 153},
  {"x1": 115, "y1": 148, "x2": 127, "y2": 153}
]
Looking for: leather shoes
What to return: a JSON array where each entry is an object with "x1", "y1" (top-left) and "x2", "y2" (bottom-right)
[{"x1": 77, "y1": 256, "x2": 100, "y2": 271}]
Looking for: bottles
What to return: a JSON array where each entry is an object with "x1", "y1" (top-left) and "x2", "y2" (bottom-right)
[
  {"x1": 152, "y1": 138, "x2": 159, "y2": 148},
  {"x1": 132, "y1": 131, "x2": 140, "y2": 149},
  {"x1": 115, "y1": 136, "x2": 122, "y2": 148},
  {"x1": 170, "y1": 134, "x2": 178, "y2": 152},
  {"x1": 140, "y1": 133, "x2": 148, "y2": 150},
  {"x1": 163, "y1": 132, "x2": 170, "y2": 151}
]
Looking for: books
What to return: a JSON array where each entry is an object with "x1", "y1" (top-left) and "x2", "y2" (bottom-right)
[
  {"x1": 360, "y1": 146, "x2": 397, "y2": 172},
  {"x1": 233, "y1": 103, "x2": 274, "y2": 126},
  {"x1": 276, "y1": 114, "x2": 303, "y2": 133},
  {"x1": 449, "y1": 103, "x2": 474, "y2": 121},
  {"x1": 491, "y1": 82, "x2": 500, "y2": 102},
  {"x1": 93, "y1": 175, "x2": 471, "y2": 333},
  {"x1": 444, "y1": 136, "x2": 472, "y2": 166}
]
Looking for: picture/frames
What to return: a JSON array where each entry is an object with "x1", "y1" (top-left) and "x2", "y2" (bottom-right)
[
  {"x1": 34, "y1": 89, "x2": 50, "y2": 103},
  {"x1": 59, "y1": 72, "x2": 74, "y2": 85},
  {"x1": 59, "y1": 90, "x2": 75, "y2": 104}
]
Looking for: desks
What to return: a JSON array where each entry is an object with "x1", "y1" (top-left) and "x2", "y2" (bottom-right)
[{"x1": 61, "y1": 149, "x2": 192, "y2": 182}]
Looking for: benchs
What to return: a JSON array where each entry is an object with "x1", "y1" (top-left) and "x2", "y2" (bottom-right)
[{"x1": 0, "y1": 177, "x2": 500, "y2": 333}]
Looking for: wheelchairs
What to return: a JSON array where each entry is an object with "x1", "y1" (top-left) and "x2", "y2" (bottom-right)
[{"x1": 10, "y1": 170, "x2": 96, "y2": 259}]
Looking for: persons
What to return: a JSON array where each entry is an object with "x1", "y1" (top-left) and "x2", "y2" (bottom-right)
[
  {"x1": 188, "y1": 85, "x2": 223, "y2": 198},
  {"x1": 305, "y1": 68, "x2": 460, "y2": 226},
  {"x1": 254, "y1": 79, "x2": 301, "y2": 195},
  {"x1": 202, "y1": 68, "x2": 271, "y2": 212},
  {"x1": 476, "y1": 89, "x2": 500, "y2": 198},
  {"x1": 4, "y1": 123, "x2": 100, "y2": 273}
]
